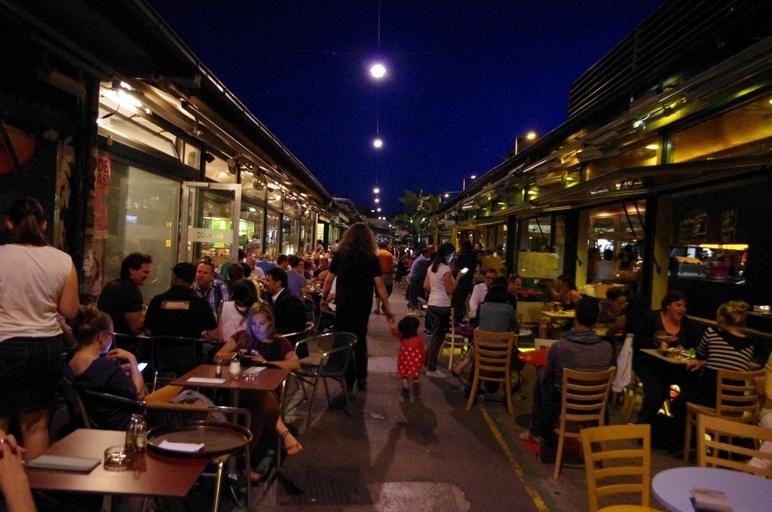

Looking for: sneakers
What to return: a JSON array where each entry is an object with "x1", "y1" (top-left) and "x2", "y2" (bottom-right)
[
  {"x1": 422, "y1": 365, "x2": 449, "y2": 380},
  {"x1": 412, "y1": 381, "x2": 421, "y2": 395},
  {"x1": 400, "y1": 383, "x2": 409, "y2": 397}
]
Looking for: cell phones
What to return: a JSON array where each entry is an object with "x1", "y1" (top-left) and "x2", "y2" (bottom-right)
[
  {"x1": 244, "y1": 351, "x2": 254, "y2": 356},
  {"x1": 137, "y1": 361, "x2": 149, "y2": 373},
  {"x1": 460, "y1": 267, "x2": 469, "y2": 273}
]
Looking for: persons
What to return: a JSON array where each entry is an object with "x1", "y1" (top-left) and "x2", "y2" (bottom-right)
[
  {"x1": 389, "y1": 236, "x2": 770, "y2": 479},
  {"x1": 372, "y1": 240, "x2": 394, "y2": 314},
  {"x1": 2, "y1": 193, "x2": 341, "y2": 511},
  {"x1": 318, "y1": 221, "x2": 395, "y2": 391}
]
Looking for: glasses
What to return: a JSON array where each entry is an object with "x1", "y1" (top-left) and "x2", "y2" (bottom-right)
[
  {"x1": 106, "y1": 330, "x2": 124, "y2": 341},
  {"x1": 613, "y1": 300, "x2": 629, "y2": 308}
]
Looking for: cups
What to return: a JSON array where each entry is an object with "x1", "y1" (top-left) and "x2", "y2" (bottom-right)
[
  {"x1": 656, "y1": 336, "x2": 668, "y2": 354},
  {"x1": 548, "y1": 300, "x2": 563, "y2": 313},
  {"x1": 229, "y1": 358, "x2": 242, "y2": 380}
]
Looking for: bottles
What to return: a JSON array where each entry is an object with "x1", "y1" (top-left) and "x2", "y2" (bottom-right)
[
  {"x1": 125, "y1": 416, "x2": 146, "y2": 450},
  {"x1": 215, "y1": 353, "x2": 223, "y2": 379}
]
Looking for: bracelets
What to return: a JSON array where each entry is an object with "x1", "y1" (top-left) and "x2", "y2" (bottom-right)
[{"x1": 320, "y1": 294, "x2": 327, "y2": 300}]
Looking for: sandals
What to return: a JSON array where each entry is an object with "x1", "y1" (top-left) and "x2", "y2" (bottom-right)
[{"x1": 279, "y1": 430, "x2": 305, "y2": 456}]
